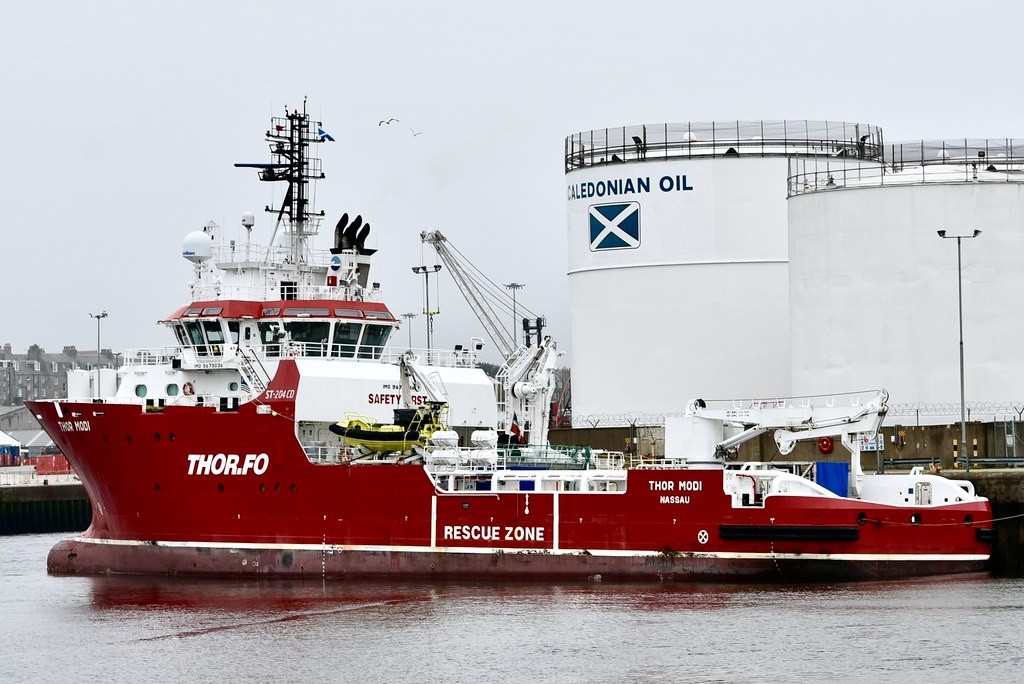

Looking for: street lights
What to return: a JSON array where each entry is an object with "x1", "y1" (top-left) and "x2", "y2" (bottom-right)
[
  {"x1": 412, "y1": 265, "x2": 442, "y2": 365},
  {"x1": 89, "y1": 311, "x2": 108, "y2": 397},
  {"x1": 937, "y1": 229, "x2": 982, "y2": 466}
]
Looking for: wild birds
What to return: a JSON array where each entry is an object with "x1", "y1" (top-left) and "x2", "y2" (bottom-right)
[
  {"x1": 379, "y1": 119, "x2": 399, "y2": 126},
  {"x1": 410, "y1": 129, "x2": 424, "y2": 136}
]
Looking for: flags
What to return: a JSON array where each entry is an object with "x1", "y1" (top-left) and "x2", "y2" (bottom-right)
[
  {"x1": 319, "y1": 129, "x2": 335, "y2": 142},
  {"x1": 510, "y1": 414, "x2": 524, "y2": 444}
]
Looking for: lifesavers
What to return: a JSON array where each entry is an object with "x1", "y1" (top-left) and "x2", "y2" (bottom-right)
[
  {"x1": 819, "y1": 437, "x2": 833, "y2": 452},
  {"x1": 184, "y1": 383, "x2": 193, "y2": 395}
]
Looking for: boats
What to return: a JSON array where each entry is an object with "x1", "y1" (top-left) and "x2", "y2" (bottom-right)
[
  {"x1": 329, "y1": 401, "x2": 449, "y2": 454},
  {"x1": 23, "y1": 93, "x2": 995, "y2": 576}
]
